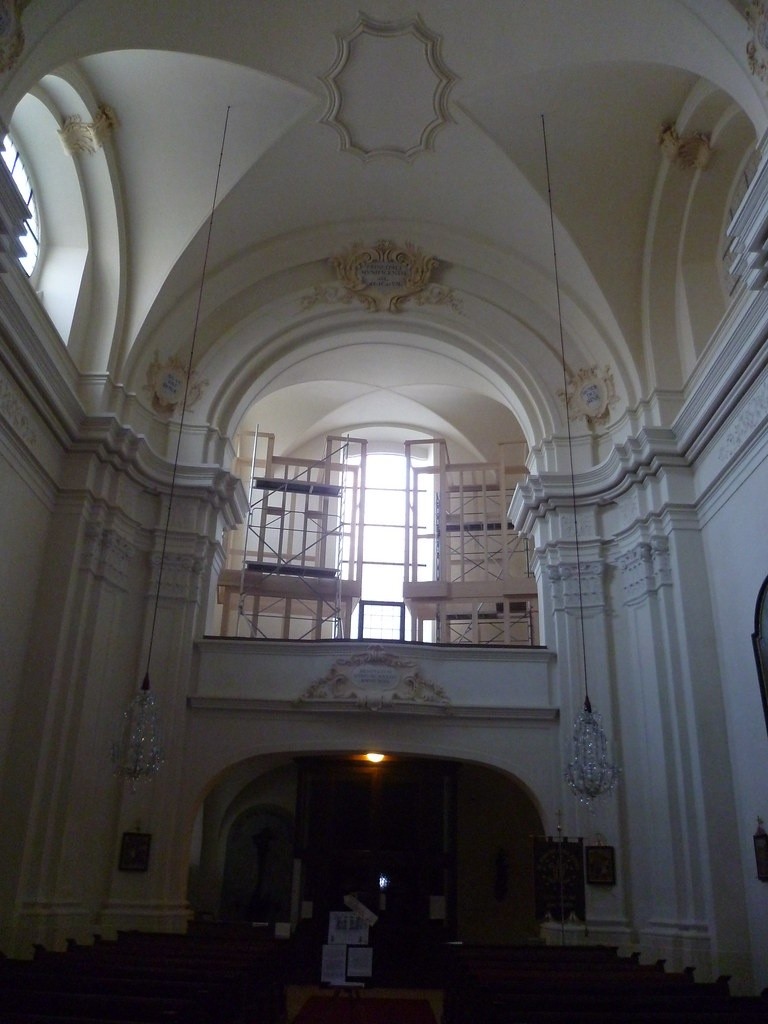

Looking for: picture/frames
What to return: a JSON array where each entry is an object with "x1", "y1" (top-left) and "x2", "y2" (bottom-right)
[
  {"x1": 586, "y1": 845, "x2": 616, "y2": 886},
  {"x1": 119, "y1": 831, "x2": 152, "y2": 871}
]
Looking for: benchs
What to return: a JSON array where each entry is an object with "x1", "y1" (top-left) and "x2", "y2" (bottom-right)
[
  {"x1": 0, "y1": 922, "x2": 313, "y2": 1024},
  {"x1": 443, "y1": 943, "x2": 768, "y2": 1024}
]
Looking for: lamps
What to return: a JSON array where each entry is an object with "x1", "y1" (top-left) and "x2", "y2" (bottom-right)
[
  {"x1": 111, "y1": 104, "x2": 230, "y2": 794},
  {"x1": 541, "y1": 114, "x2": 619, "y2": 806}
]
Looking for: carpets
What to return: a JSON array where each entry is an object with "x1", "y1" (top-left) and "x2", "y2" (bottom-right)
[{"x1": 290, "y1": 995, "x2": 438, "y2": 1024}]
